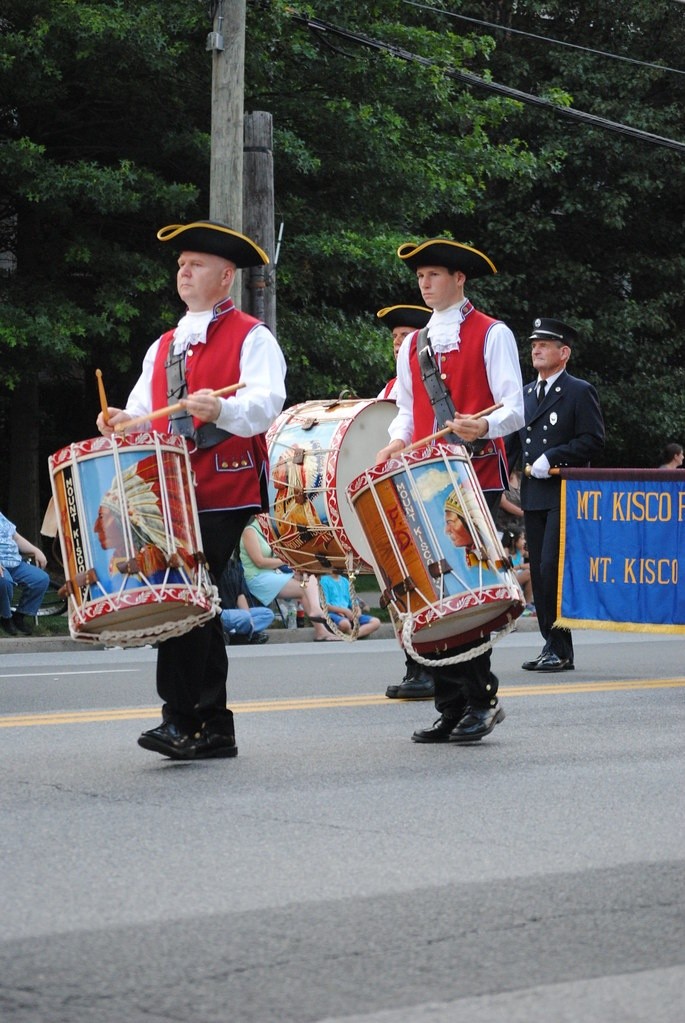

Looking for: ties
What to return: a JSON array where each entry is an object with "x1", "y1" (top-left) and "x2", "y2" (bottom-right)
[{"x1": 538, "y1": 379, "x2": 547, "y2": 406}]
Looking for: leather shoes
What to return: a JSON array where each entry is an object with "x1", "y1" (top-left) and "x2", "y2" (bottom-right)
[
  {"x1": 386, "y1": 673, "x2": 434, "y2": 698},
  {"x1": 410, "y1": 712, "x2": 483, "y2": 743},
  {"x1": 449, "y1": 702, "x2": 506, "y2": 742},
  {"x1": 522, "y1": 653, "x2": 575, "y2": 673},
  {"x1": 135, "y1": 716, "x2": 238, "y2": 759}
]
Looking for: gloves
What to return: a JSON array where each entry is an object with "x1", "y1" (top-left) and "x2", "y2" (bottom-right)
[{"x1": 531, "y1": 453, "x2": 552, "y2": 480}]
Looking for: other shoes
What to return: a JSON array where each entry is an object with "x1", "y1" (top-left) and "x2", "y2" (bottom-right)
[
  {"x1": 252, "y1": 632, "x2": 269, "y2": 644},
  {"x1": 11, "y1": 612, "x2": 32, "y2": 636},
  {"x1": 0, "y1": 617, "x2": 18, "y2": 636},
  {"x1": 524, "y1": 604, "x2": 536, "y2": 617},
  {"x1": 229, "y1": 633, "x2": 254, "y2": 645}
]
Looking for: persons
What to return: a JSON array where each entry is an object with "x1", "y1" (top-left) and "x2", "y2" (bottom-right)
[
  {"x1": 378, "y1": 305, "x2": 437, "y2": 699},
  {"x1": 376, "y1": 239, "x2": 524, "y2": 743},
  {"x1": 0, "y1": 443, "x2": 684, "y2": 641},
  {"x1": 97, "y1": 220, "x2": 287, "y2": 762},
  {"x1": 498, "y1": 316, "x2": 609, "y2": 672}
]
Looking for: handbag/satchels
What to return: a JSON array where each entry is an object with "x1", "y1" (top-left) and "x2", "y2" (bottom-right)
[{"x1": 279, "y1": 564, "x2": 293, "y2": 574}]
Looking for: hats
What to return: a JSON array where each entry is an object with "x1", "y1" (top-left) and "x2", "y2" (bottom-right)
[
  {"x1": 375, "y1": 305, "x2": 435, "y2": 331},
  {"x1": 396, "y1": 238, "x2": 498, "y2": 279},
  {"x1": 527, "y1": 318, "x2": 581, "y2": 349},
  {"x1": 155, "y1": 221, "x2": 270, "y2": 268}
]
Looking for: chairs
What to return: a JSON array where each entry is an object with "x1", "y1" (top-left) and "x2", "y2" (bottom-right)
[{"x1": 236, "y1": 551, "x2": 289, "y2": 627}]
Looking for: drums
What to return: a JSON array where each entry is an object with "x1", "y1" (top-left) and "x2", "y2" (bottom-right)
[
  {"x1": 255, "y1": 397, "x2": 400, "y2": 577},
  {"x1": 342, "y1": 444, "x2": 526, "y2": 657},
  {"x1": 46, "y1": 430, "x2": 219, "y2": 649}
]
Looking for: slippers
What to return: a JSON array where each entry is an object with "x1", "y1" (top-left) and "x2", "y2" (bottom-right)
[
  {"x1": 313, "y1": 636, "x2": 344, "y2": 642},
  {"x1": 308, "y1": 612, "x2": 327, "y2": 623}
]
